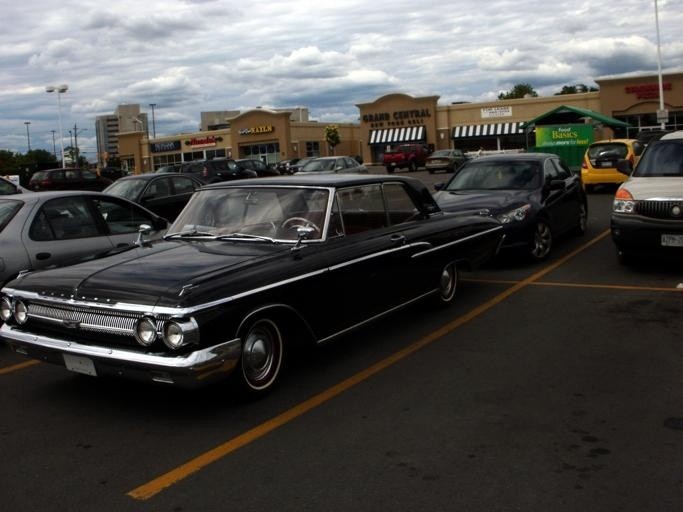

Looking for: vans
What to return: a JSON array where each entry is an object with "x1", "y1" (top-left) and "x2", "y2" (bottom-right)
[{"x1": 608, "y1": 130, "x2": 682, "y2": 273}]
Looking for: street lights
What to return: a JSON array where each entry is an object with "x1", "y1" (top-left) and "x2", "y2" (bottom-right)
[
  {"x1": 45, "y1": 82, "x2": 69, "y2": 169},
  {"x1": 149, "y1": 103, "x2": 158, "y2": 137},
  {"x1": 49, "y1": 130, "x2": 57, "y2": 154},
  {"x1": 24, "y1": 122, "x2": 31, "y2": 149}
]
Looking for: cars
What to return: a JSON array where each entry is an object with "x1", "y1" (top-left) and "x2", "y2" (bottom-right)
[
  {"x1": 431, "y1": 153, "x2": 589, "y2": 265},
  {"x1": 0, "y1": 191, "x2": 174, "y2": 291},
  {"x1": 0, "y1": 176, "x2": 30, "y2": 194},
  {"x1": 1, "y1": 172, "x2": 509, "y2": 403},
  {"x1": 28, "y1": 170, "x2": 112, "y2": 192},
  {"x1": 579, "y1": 135, "x2": 645, "y2": 192},
  {"x1": 103, "y1": 173, "x2": 211, "y2": 224},
  {"x1": 156, "y1": 158, "x2": 304, "y2": 183},
  {"x1": 294, "y1": 156, "x2": 366, "y2": 177},
  {"x1": 424, "y1": 148, "x2": 467, "y2": 175}
]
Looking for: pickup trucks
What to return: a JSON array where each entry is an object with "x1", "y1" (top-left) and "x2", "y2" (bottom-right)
[{"x1": 382, "y1": 144, "x2": 432, "y2": 173}]
